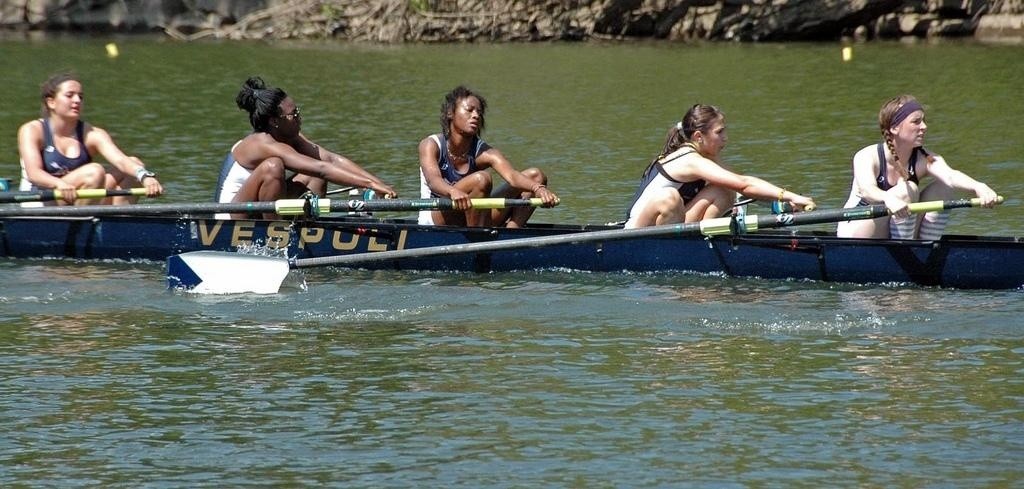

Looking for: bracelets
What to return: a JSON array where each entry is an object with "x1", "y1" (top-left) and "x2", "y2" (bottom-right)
[
  {"x1": 778, "y1": 188, "x2": 785, "y2": 202},
  {"x1": 531, "y1": 182, "x2": 545, "y2": 194}
]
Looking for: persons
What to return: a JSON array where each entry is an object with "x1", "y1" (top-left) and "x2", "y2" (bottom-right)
[
  {"x1": 835, "y1": 94, "x2": 1000, "y2": 243},
  {"x1": 621, "y1": 102, "x2": 818, "y2": 231},
  {"x1": 414, "y1": 86, "x2": 558, "y2": 229},
  {"x1": 213, "y1": 73, "x2": 398, "y2": 224},
  {"x1": 13, "y1": 70, "x2": 164, "y2": 208}
]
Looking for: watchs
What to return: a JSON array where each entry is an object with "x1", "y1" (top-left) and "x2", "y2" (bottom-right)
[{"x1": 136, "y1": 170, "x2": 157, "y2": 184}]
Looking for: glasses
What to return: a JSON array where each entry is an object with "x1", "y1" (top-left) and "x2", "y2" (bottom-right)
[{"x1": 280, "y1": 107, "x2": 301, "y2": 122}]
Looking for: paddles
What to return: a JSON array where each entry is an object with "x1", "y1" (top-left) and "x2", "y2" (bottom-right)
[{"x1": 167, "y1": 196, "x2": 1002, "y2": 294}]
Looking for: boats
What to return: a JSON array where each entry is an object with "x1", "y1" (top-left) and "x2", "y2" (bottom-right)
[{"x1": 0, "y1": 177, "x2": 1024, "y2": 291}]
